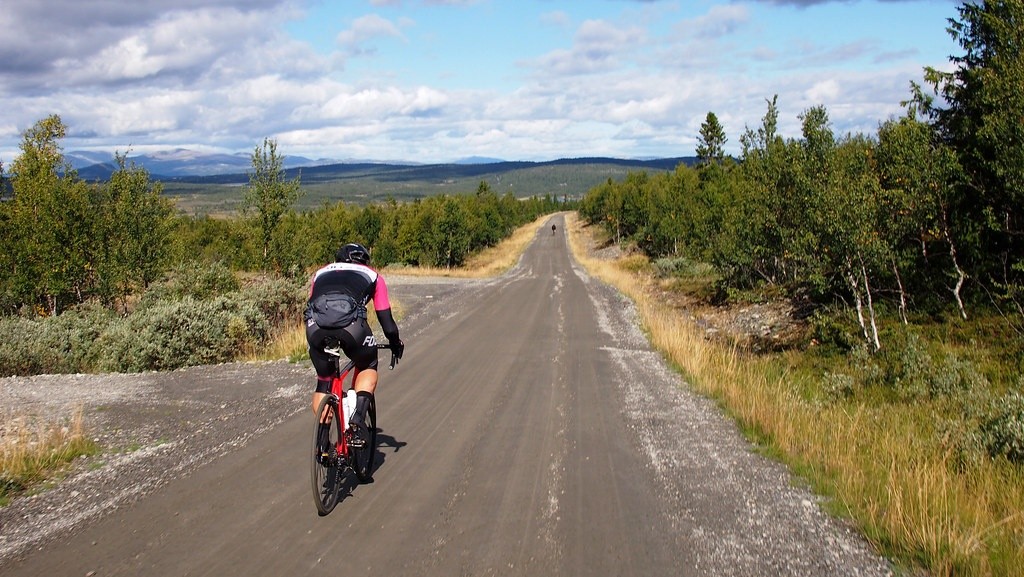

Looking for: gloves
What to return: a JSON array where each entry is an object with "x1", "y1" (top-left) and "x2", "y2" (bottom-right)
[{"x1": 389, "y1": 340, "x2": 404, "y2": 359}]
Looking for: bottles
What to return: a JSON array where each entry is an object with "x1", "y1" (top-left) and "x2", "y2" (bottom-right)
[
  {"x1": 347, "y1": 388, "x2": 357, "y2": 417},
  {"x1": 342, "y1": 392, "x2": 350, "y2": 433}
]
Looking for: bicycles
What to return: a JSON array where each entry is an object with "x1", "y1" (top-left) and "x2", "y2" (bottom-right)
[{"x1": 310, "y1": 337, "x2": 400, "y2": 516}]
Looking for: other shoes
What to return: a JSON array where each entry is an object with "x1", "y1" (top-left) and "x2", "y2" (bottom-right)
[
  {"x1": 319, "y1": 451, "x2": 330, "y2": 468},
  {"x1": 349, "y1": 408, "x2": 369, "y2": 448}
]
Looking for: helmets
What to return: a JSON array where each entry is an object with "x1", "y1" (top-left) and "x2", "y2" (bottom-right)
[{"x1": 335, "y1": 243, "x2": 370, "y2": 266}]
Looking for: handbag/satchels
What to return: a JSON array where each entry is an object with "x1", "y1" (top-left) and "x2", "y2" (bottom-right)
[{"x1": 303, "y1": 291, "x2": 367, "y2": 329}]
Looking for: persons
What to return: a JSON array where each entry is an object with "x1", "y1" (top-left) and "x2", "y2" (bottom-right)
[
  {"x1": 306, "y1": 243, "x2": 404, "y2": 467},
  {"x1": 552, "y1": 224, "x2": 556, "y2": 235}
]
